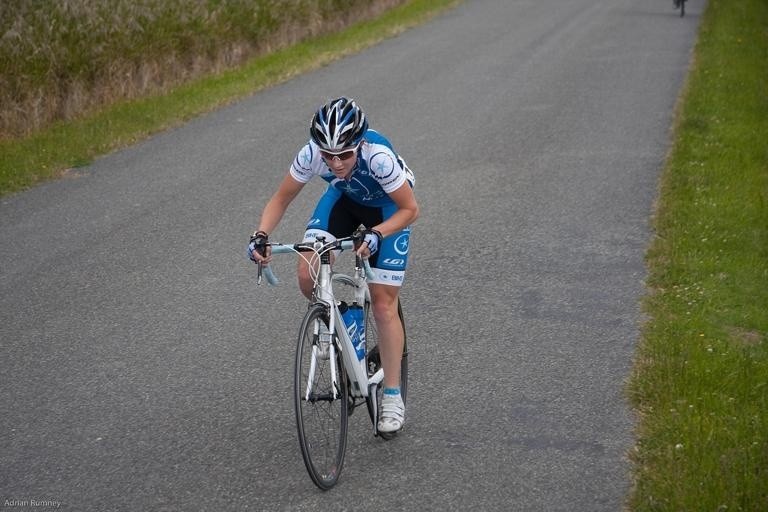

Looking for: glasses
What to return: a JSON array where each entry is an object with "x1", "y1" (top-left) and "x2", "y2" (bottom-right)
[{"x1": 318, "y1": 142, "x2": 361, "y2": 161}]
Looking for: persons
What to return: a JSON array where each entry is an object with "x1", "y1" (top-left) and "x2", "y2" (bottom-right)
[{"x1": 245, "y1": 95, "x2": 421, "y2": 436}]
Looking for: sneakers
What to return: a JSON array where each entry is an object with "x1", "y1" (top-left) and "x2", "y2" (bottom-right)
[
  {"x1": 377, "y1": 392, "x2": 406, "y2": 433},
  {"x1": 316, "y1": 320, "x2": 331, "y2": 360}
]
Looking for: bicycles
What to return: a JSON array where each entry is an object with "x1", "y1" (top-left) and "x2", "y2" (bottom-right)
[{"x1": 256, "y1": 224, "x2": 408, "y2": 492}]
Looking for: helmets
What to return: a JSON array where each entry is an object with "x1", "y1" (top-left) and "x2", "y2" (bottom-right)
[{"x1": 309, "y1": 96, "x2": 369, "y2": 152}]
